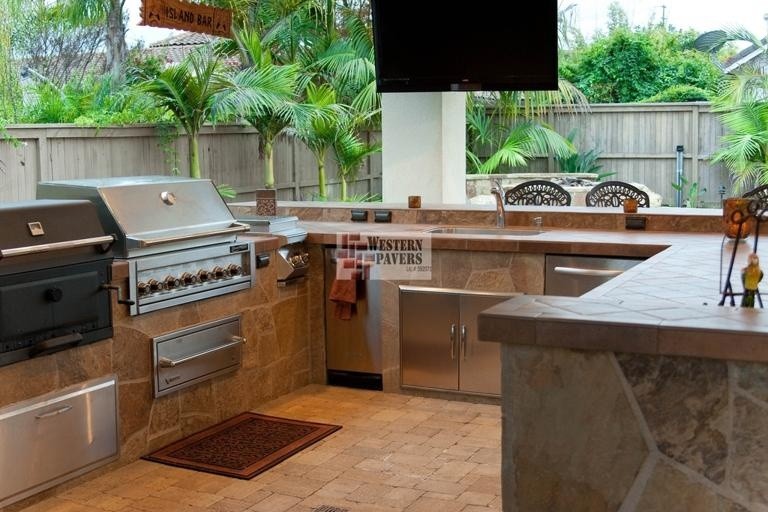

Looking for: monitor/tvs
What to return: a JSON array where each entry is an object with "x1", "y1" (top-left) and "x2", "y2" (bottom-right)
[{"x1": 370, "y1": 0, "x2": 559, "y2": 93}]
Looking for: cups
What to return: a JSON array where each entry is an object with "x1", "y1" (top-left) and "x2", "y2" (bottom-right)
[
  {"x1": 722, "y1": 197, "x2": 755, "y2": 239},
  {"x1": 624, "y1": 198, "x2": 638, "y2": 213},
  {"x1": 408, "y1": 196, "x2": 421, "y2": 208}
]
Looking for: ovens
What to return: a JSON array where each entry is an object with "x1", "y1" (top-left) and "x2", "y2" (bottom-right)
[{"x1": 36, "y1": 175, "x2": 256, "y2": 317}]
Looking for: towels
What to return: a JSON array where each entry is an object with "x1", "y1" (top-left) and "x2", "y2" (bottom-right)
[{"x1": 330, "y1": 257, "x2": 361, "y2": 322}]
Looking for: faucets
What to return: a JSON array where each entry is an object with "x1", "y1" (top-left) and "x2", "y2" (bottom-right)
[{"x1": 490, "y1": 178, "x2": 505, "y2": 227}]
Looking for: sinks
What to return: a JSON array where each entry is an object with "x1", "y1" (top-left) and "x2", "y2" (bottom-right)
[{"x1": 422, "y1": 226, "x2": 554, "y2": 236}]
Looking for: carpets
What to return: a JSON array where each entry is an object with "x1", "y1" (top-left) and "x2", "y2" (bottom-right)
[{"x1": 139, "y1": 410, "x2": 342, "y2": 480}]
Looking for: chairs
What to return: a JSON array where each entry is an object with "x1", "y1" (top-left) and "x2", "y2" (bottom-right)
[{"x1": 506, "y1": 179, "x2": 650, "y2": 208}]
